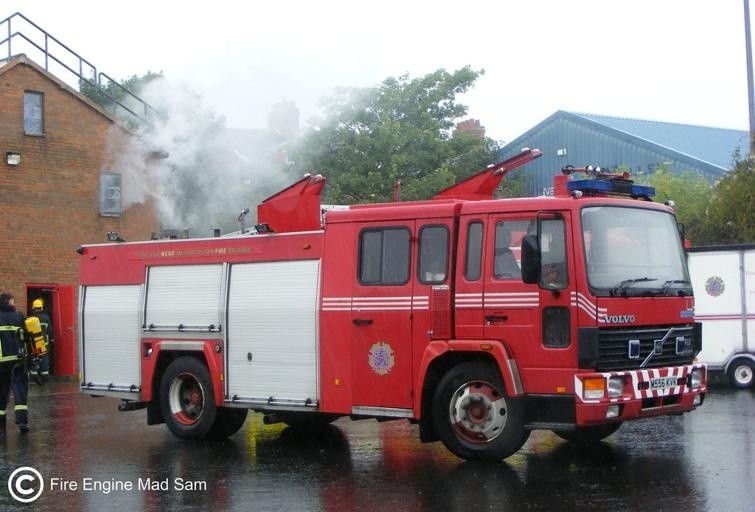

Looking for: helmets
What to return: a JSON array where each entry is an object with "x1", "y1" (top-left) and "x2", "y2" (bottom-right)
[{"x1": 31, "y1": 298, "x2": 44, "y2": 310}]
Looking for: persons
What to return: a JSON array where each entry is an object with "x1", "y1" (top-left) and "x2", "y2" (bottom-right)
[
  {"x1": 29, "y1": 299, "x2": 53, "y2": 385},
  {"x1": 0, "y1": 293, "x2": 32, "y2": 433}
]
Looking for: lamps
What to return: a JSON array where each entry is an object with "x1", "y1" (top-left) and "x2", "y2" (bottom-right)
[{"x1": 3, "y1": 150, "x2": 24, "y2": 168}]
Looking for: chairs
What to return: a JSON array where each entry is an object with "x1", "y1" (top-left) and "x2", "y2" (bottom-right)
[{"x1": 496, "y1": 228, "x2": 521, "y2": 278}]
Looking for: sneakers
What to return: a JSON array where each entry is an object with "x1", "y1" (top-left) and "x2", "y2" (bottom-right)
[
  {"x1": 18, "y1": 422, "x2": 30, "y2": 431},
  {"x1": 0, "y1": 427, "x2": 7, "y2": 438}
]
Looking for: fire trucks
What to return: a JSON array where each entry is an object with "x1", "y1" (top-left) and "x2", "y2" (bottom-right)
[{"x1": 75, "y1": 146, "x2": 707, "y2": 461}]
